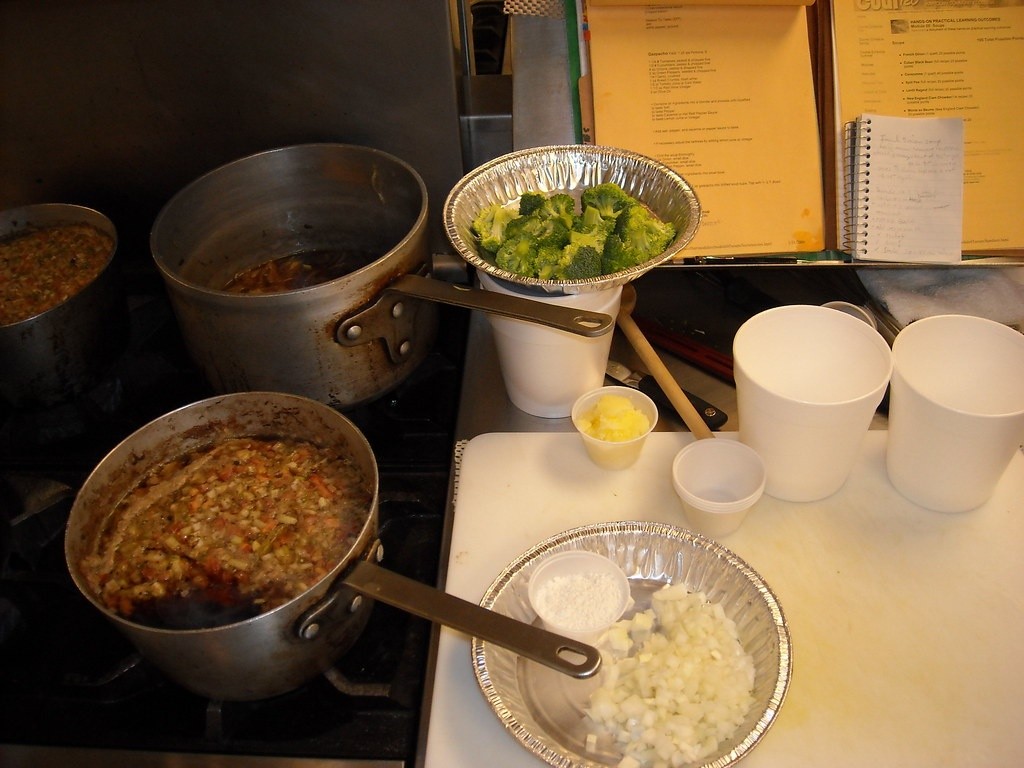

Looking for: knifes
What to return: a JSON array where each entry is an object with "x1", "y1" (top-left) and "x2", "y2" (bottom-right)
[{"x1": 605, "y1": 360, "x2": 730, "y2": 428}]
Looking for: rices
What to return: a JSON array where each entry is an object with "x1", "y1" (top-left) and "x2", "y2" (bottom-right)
[{"x1": 535, "y1": 572, "x2": 620, "y2": 631}]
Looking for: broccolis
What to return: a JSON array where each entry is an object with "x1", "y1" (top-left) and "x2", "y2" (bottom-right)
[{"x1": 474, "y1": 184, "x2": 675, "y2": 281}]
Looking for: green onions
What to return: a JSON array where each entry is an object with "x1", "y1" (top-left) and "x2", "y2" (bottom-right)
[{"x1": 584, "y1": 584, "x2": 755, "y2": 768}]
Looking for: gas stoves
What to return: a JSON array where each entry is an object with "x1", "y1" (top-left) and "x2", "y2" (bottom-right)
[{"x1": 2, "y1": 163, "x2": 485, "y2": 768}]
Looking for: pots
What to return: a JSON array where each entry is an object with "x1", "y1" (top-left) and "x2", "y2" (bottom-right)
[
  {"x1": 65, "y1": 391, "x2": 602, "y2": 705},
  {"x1": 149, "y1": 143, "x2": 613, "y2": 412}
]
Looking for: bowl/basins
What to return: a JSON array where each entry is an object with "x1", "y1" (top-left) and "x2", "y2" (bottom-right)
[
  {"x1": 442, "y1": 144, "x2": 701, "y2": 296},
  {"x1": 470, "y1": 521, "x2": 791, "y2": 767},
  {"x1": 1, "y1": 202, "x2": 117, "y2": 338}
]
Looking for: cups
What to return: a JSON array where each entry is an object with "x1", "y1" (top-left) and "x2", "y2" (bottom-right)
[
  {"x1": 571, "y1": 386, "x2": 660, "y2": 468},
  {"x1": 735, "y1": 305, "x2": 1024, "y2": 512},
  {"x1": 672, "y1": 437, "x2": 766, "y2": 533},
  {"x1": 482, "y1": 273, "x2": 621, "y2": 418},
  {"x1": 528, "y1": 551, "x2": 632, "y2": 641}
]
